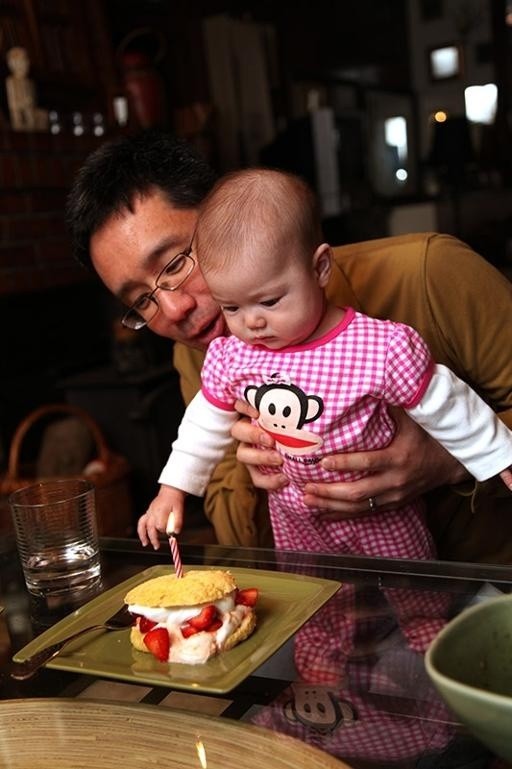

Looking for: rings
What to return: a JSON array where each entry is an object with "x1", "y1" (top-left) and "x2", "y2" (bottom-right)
[{"x1": 368, "y1": 496, "x2": 376, "y2": 513}]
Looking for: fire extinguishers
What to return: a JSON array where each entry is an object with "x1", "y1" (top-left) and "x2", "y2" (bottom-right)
[{"x1": 117, "y1": 49, "x2": 167, "y2": 130}]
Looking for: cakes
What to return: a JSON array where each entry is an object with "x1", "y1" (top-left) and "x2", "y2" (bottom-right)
[{"x1": 123, "y1": 569, "x2": 260, "y2": 666}]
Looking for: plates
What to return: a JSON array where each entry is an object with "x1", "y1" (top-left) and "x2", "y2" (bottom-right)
[{"x1": 11, "y1": 564, "x2": 344, "y2": 696}]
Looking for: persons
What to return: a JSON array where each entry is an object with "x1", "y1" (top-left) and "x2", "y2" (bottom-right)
[
  {"x1": 61, "y1": 131, "x2": 511, "y2": 573},
  {"x1": 132, "y1": 167, "x2": 511, "y2": 689}
]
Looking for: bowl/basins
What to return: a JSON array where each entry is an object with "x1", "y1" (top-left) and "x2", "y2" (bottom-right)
[{"x1": 423, "y1": 594, "x2": 512, "y2": 761}]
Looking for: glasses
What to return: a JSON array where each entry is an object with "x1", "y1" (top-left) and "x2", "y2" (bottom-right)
[{"x1": 121, "y1": 227, "x2": 196, "y2": 330}]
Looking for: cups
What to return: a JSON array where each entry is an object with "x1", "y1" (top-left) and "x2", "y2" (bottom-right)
[{"x1": 9, "y1": 477, "x2": 104, "y2": 598}]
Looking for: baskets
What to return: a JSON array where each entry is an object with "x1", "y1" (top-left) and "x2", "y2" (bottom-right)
[{"x1": 0, "y1": 405, "x2": 133, "y2": 539}]
